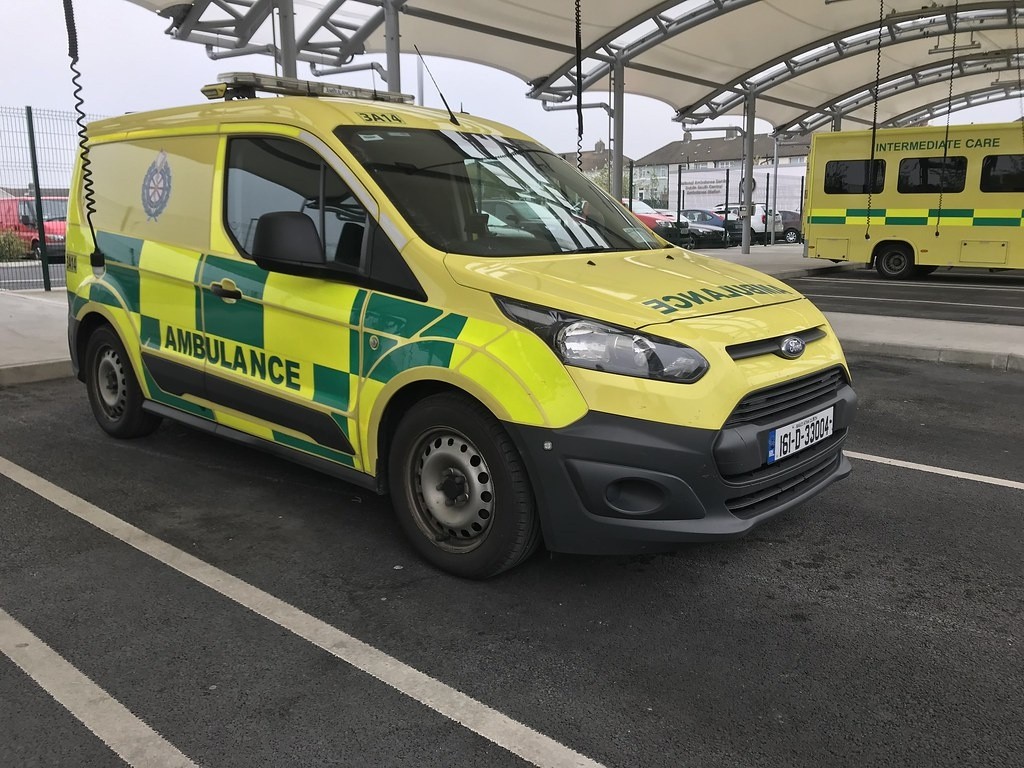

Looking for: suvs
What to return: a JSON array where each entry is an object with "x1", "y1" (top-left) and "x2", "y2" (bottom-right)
[{"x1": 713, "y1": 202, "x2": 784, "y2": 245}]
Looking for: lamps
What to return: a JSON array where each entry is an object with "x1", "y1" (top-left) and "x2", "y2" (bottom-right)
[
  {"x1": 991, "y1": 70, "x2": 1024, "y2": 85},
  {"x1": 927, "y1": 31, "x2": 981, "y2": 55}
]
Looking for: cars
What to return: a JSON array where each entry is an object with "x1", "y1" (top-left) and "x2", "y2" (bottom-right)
[
  {"x1": 654, "y1": 208, "x2": 732, "y2": 248},
  {"x1": 477, "y1": 210, "x2": 535, "y2": 249},
  {"x1": 769, "y1": 209, "x2": 801, "y2": 244},
  {"x1": 678, "y1": 210, "x2": 752, "y2": 245},
  {"x1": 476, "y1": 200, "x2": 587, "y2": 245},
  {"x1": 581, "y1": 197, "x2": 693, "y2": 245}
]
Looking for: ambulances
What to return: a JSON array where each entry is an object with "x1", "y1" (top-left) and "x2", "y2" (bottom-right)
[
  {"x1": 65, "y1": 72, "x2": 856, "y2": 579},
  {"x1": 801, "y1": 122, "x2": 1024, "y2": 278}
]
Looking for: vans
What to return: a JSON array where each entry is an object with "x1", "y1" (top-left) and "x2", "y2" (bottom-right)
[{"x1": 0, "y1": 197, "x2": 68, "y2": 260}]
